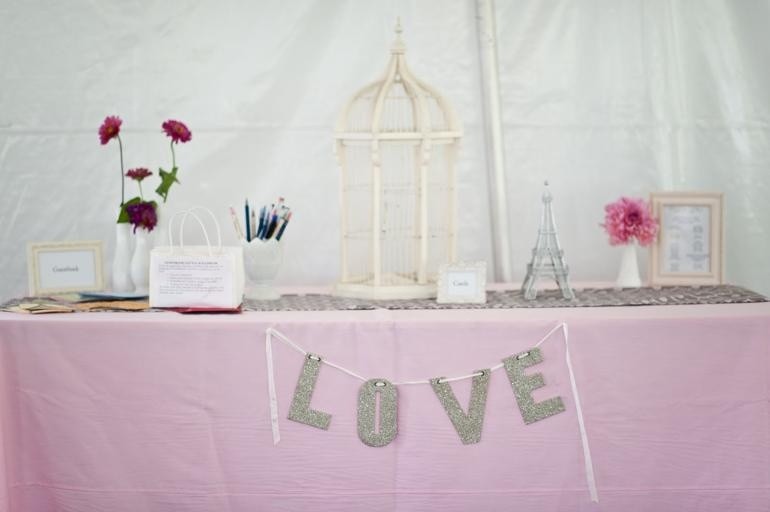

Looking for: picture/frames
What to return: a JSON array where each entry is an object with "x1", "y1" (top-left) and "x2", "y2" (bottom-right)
[
  {"x1": 647, "y1": 191, "x2": 725, "y2": 287},
  {"x1": 436, "y1": 263, "x2": 487, "y2": 305},
  {"x1": 27, "y1": 240, "x2": 104, "y2": 298}
]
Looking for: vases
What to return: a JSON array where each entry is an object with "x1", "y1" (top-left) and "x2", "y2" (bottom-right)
[
  {"x1": 129, "y1": 226, "x2": 154, "y2": 294},
  {"x1": 112, "y1": 222, "x2": 133, "y2": 291},
  {"x1": 616, "y1": 242, "x2": 644, "y2": 290}
]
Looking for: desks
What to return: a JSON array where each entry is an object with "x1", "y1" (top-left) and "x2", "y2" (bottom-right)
[{"x1": 1, "y1": 292, "x2": 770, "y2": 512}]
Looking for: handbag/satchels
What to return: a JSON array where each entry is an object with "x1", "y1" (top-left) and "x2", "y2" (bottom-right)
[{"x1": 148, "y1": 208, "x2": 243, "y2": 313}]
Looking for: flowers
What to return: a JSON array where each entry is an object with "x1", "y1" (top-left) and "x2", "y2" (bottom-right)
[
  {"x1": 116, "y1": 117, "x2": 192, "y2": 233},
  {"x1": 604, "y1": 194, "x2": 657, "y2": 246},
  {"x1": 98, "y1": 114, "x2": 126, "y2": 222}
]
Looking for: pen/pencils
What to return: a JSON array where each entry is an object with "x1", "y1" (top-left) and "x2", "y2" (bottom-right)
[{"x1": 229, "y1": 196, "x2": 292, "y2": 242}]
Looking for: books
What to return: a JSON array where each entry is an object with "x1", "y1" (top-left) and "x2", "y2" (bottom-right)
[{"x1": 0, "y1": 291, "x2": 149, "y2": 314}]
